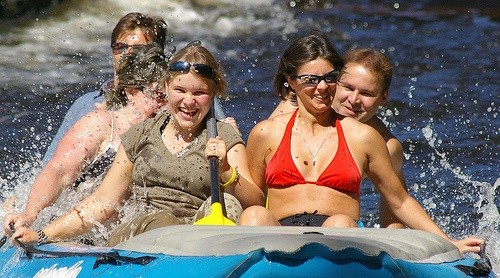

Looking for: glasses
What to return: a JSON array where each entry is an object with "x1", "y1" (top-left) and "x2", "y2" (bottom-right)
[
  {"x1": 293, "y1": 68, "x2": 339, "y2": 84},
  {"x1": 110, "y1": 42, "x2": 144, "y2": 52},
  {"x1": 167, "y1": 61, "x2": 216, "y2": 83}
]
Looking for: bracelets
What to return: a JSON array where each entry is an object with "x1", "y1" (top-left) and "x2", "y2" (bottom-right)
[
  {"x1": 74, "y1": 207, "x2": 85, "y2": 227},
  {"x1": 220, "y1": 166, "x2": 239, "y2": 188},
  {"x1": 37, "y1": 229, "x2": 48, "y2": 245}
]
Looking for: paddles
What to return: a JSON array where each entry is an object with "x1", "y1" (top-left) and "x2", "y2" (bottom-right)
[{"x1": 192, "y1": 98, "x2": 236, "y2": 225}]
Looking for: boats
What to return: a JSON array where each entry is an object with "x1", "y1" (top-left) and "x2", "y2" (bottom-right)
[{"x1": 0, "y1": 205, "x2": 500, "y2": 278}]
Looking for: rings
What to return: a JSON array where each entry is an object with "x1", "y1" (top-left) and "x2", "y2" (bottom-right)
[
  {"x1": 216, "y1": 143, "x2": 218, "y2": 146},
  {"x1": 214, "y1": 147, "x2": 215, "y2": 150},
  {"x1": 213, "y1": 145, "x2": 215, "y2": 147}
]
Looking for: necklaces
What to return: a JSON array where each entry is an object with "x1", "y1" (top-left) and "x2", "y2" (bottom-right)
[{"x1": 296, "y1": 125, "x2": 331, "y2": 166}]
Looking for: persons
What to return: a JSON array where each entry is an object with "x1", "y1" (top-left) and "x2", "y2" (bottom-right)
[
  {"x1": 268, "y1": 47, "x2": 407, "y2": 230},
  {"x1": 3, "y1": 42, "x2": 243, "y2": 237},
  {"x1": 238, "y1": 34, "x2": 488, "y2": 255},
  {"x1": 12, "y1": 46, "x2": 265, "y2": 254},
  {"x1": 6, "y1": 13, "x2": 169, "y2": 213}
]
